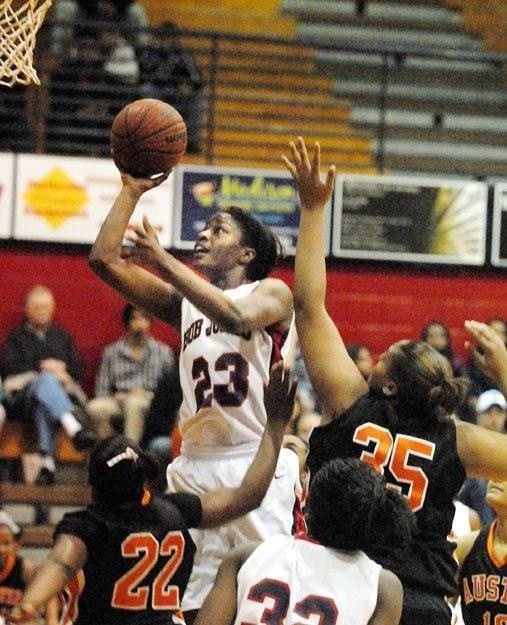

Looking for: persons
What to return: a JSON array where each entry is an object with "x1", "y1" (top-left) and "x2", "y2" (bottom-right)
[
  {"x1": 279, "y1": 133, "x2": 506, "y2": 625},
  {"x1": 283, "y1": 315, "x2": 507, "y2": 624},
  {"x1": 139, "y1": 19, "x2": 205, "y2": 152},
  {"x1": 5, "y1": 359, "x2": 300, "y2": 625},
  {"x1": 0, "y1": 511, "x2": 60, "y2": 625},
  {"x1": 84, "y1": 152, "x2": 297, "y2": 625},
  {"x1": 1, "y1": 282, "x2": 99, "y2": 495},
  {"x1": 36, "y1": 0, "x2": 151, "y2": 160},
  {"x1": 194, "y1": 454, "x2": 417, "y2": 625},
  {"x1": 81, "y1": 300, "x2": 175, "y2": 445}
]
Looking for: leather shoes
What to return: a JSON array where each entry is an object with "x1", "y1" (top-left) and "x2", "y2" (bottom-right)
[
  {"x1": 36, "y1": 467, "x2": 57, "y2": 486},
  {"x1": 73, "y1": 429, "x2": 98, "y2": 451}
]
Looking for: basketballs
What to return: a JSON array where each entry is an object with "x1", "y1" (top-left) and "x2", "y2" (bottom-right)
[{"x1": 111, "y1": 97, "x2": 188, "y2": 177}]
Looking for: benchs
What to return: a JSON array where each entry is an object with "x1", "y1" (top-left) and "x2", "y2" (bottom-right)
[
  {"x1": 0, "y1": 0, "x2": 507, "y2": 176},
  {"x1": 0, "y1": 415, "x2": 181, "y2": 547}
]
